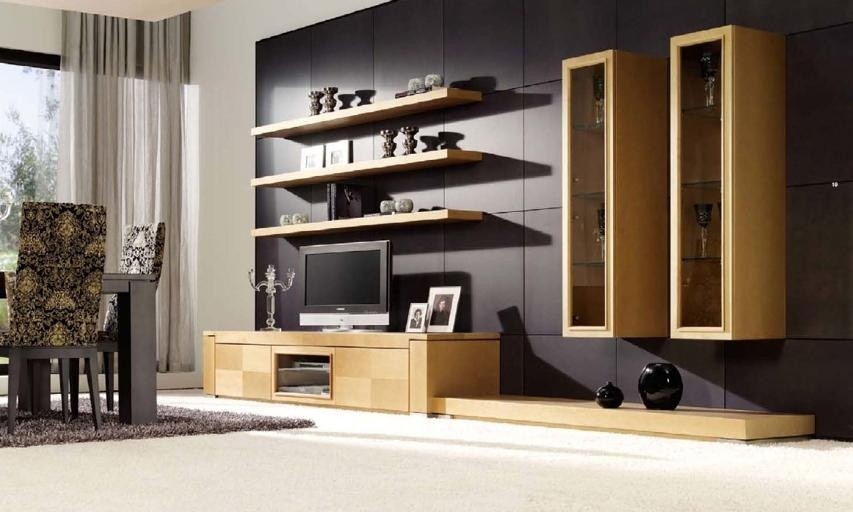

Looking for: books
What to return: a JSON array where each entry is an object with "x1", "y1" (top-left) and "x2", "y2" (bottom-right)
[{"x1": 327, "y1": 183, "x2": 362, "y2": 220}]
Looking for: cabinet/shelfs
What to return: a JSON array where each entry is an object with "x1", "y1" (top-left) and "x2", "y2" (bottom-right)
[
  {"x1": 335, "y1": 347, "x2": 409, "y2": 415},
  {"x1": 561, "y1": 49, "x2": 668, "y2": 337},
  {"x1": 215, "y1": 344, "x2": 271, "y2": 400},
  {"x1": 251, "y1": 88, "x2": 484, "y2": 238},
  {"x1": 271, "y1": 346, "x2": 335, "y2": 409},
  {"x1": 669, "y1": 24, "x2": 786, "y2": 341}
]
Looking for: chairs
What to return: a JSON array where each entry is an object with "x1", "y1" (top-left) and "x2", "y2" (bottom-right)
[{"x1": 0, "y1": 201, "x2": 164, "y2": 434}]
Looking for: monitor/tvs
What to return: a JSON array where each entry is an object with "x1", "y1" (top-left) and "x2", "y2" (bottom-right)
[{"x1": 299, "y1": 240, "x2": 391, "y2": 332}]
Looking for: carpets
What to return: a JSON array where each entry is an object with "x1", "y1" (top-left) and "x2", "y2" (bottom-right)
[{"x1": 0, "y1": 397, "x2": 315, "y2": 446}]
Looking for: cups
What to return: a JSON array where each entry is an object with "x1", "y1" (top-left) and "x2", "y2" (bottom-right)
[
  {"x1": 380, "y1": 199, "x2": 412, "y2": 214},
  {"x1": 278, "y1": 213, "x2": 308, "y2": 225},
  {"x1": 407, "y1": 73, "x2": 444, "y2": 93}
]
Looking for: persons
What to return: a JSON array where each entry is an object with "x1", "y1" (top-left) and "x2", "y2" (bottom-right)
[
  {"x1": 410, "y1": 309, "x2": 422, "y2": 328},
  {"x1": 433, "y1": 298, "x2": 450, "y2": 324}
]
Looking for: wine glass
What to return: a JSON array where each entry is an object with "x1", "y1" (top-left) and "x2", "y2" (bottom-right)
[
  {"x1": 700, "y1": 50, "x2": 718, "y2": 106},
  {"x1": 591, "y1": 74, "x2": 603, "y2": 123},
  {"x1": 694, "y1": 203, "x2": 714, "y2": 258}
]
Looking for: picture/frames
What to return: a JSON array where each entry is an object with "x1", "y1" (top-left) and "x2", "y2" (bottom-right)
[
  {"x1": 405, "y1": 303, "x2": 428, "y2": 333},
  {"x1": 428, "y1": 286, "x2": 462, "y2": 332}
]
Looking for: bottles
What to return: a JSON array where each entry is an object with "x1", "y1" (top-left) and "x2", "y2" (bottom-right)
[
  {"x1": 639, "y1": 361, "x2": 682, "y2": 411},
  {"x1": 596, "y1": 381, "x2": 624, "y2": 410}
]
